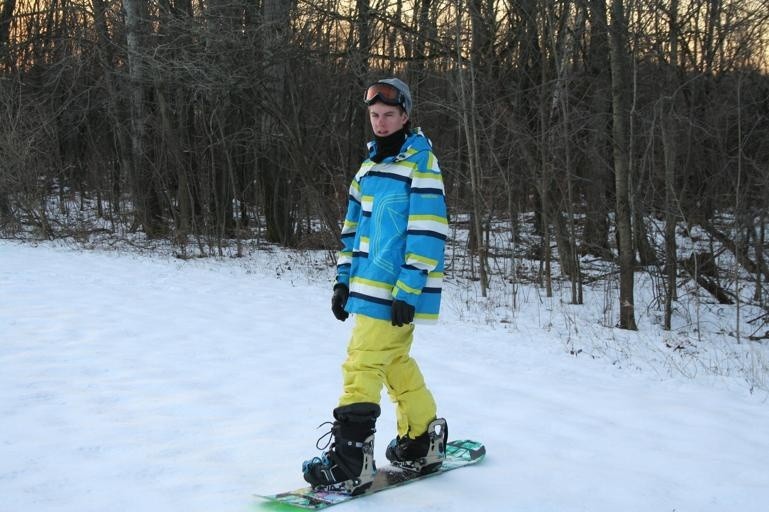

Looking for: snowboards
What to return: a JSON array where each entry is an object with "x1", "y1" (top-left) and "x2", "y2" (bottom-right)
[{"x1": 252, "y1": 418, "x2": 486, "y2": 510}]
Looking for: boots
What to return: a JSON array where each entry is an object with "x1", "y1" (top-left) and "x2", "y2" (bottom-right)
[
  {"x1": 385, "y1": 430, "x2": 430, "y2": 462},
  {"x1": 302, "y1": 403, "x2": 381, "y2": 486}
]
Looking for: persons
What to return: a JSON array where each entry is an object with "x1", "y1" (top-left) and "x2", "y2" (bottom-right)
[{"x1": 302, "y1": 77, "x2": 452, "y2": 496}]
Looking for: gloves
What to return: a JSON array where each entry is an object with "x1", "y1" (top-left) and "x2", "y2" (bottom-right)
[
  {"x1": 391, "y1": 298, "x2": 415, "y2": 327},
  {"x1": 331, "y1": 284, "x2": 349, "y2": 321}
]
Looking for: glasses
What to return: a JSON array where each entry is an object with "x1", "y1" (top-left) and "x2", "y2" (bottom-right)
[{"x1": 363, "y1": 81, "x2": 406, "y2": 106}]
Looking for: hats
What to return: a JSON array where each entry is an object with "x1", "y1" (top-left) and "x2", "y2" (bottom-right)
[{"x1": 378, "y1": 79, "x2": 412, "y2": 115}]
